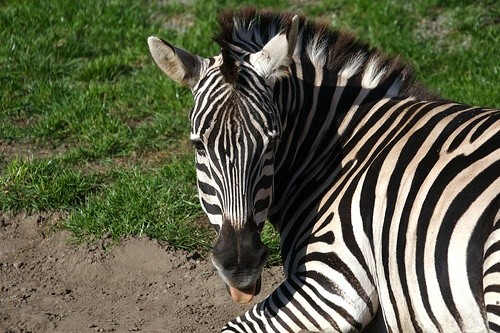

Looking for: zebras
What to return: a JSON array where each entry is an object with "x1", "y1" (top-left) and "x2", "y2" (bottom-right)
[{"x1": 146, "y1": 6, "x2": 500, "y2": 333}]
